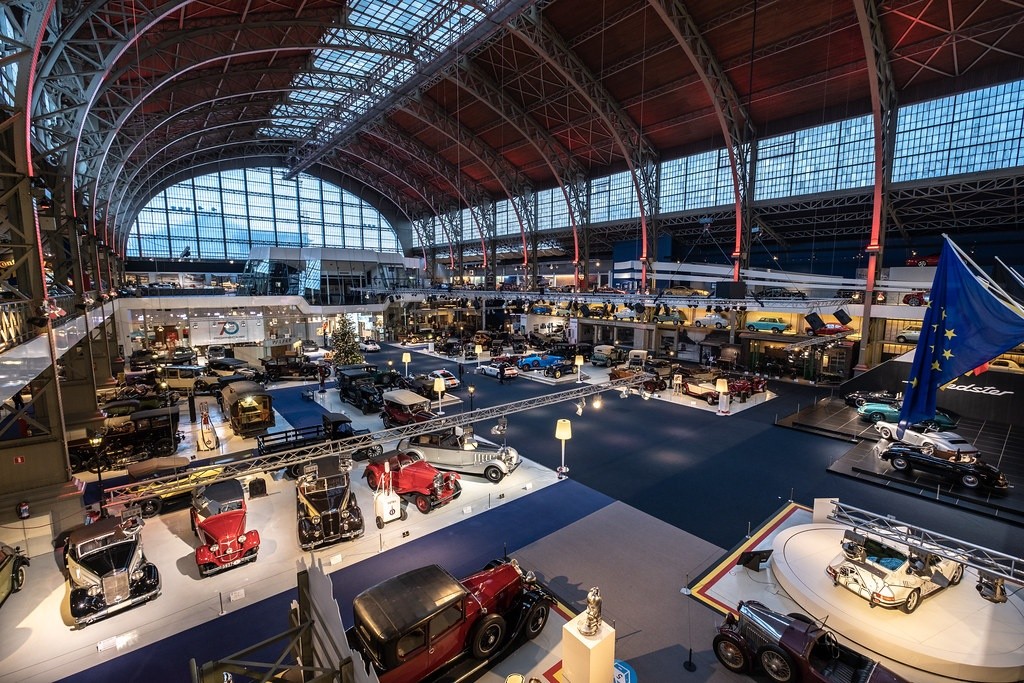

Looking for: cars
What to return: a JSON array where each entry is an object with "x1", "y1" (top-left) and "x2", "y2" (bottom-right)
[
  {"x1": 901, "y1": 289, "x2": 932, "y2": 307},
  {"x1": 38, "y1": 278, "x2": 379, "y2": 631},
  {"x1": 844, "y1": 388, "x2": 904, "y2": 408},
  {"x1": 0, "y1": 541, "x2": 30, "y2": 609},
  {"x1": 345, "y1": 543, "x2": 559, "y2": 682},
  {"x1": 878, "y1": 441, "x2": 1011, "y2": 495},
  {"x1": 906, "y1": 243, "x2": 977, "y2": 266},
  {"x1": 989, "y1": 359, "x2": 1024, "y2": 375},
  {"x1": 713, "y1": 600, "x2": 909, "y2": 683},
  {"x1": 379, "y1": 276, "x2": 888, "y2": 405},
  {"x1": 834, "y1": 538, "x2": 968, "y2": 614},
  {"x1": 874, "y1": 419, "x2": 982, "y2": 461},
  {"x1": 381, "y1": 388, "x2": 440, "y2": 429},
  {"x1": 895, "y1": 324, "x2": 923, "y2": 343},
  {"x1": 396, "y1": 426, "x2": 522, "y2": 484},
  {"x1": 362, "y1": 449, "x2": 461, "y2": 515},
  {"x1": 857, "y1": 399, "x2": 961, "y2": 432}
]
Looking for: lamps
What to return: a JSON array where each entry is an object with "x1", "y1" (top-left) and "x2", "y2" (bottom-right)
[
  {"x1": 434, "y1": 378, "x2": 446, "y2": 415},
  {"x1": 904, "y1": 545, "x2": 934, "y2": 578},
  {"x1": 653, "y1": 304, "x2": 661, "y2": 317},
  {"x1": 555, "y1": 419, "x2": 572, "y2": 472},
  {"x1": 975, "y1": 569, "x2": 1008, "y2": 604},
  {"x1": 593, "y1": 392, "x2": 602, "y2": 409},
  {"x1": 717, "y1": 379, "x2": 728, "y2": 395},
  {"x1": 574, "y1": 355, "x2": 584, "y2": 384},
  {"x1": 852, "y1": 291, "x2": 860, "y2": 299},
  {"x1": 491, "y1": 414, "x2": 508, "y2": 435},
  {"x1": 575, "y1": 397, "x2": 586, "y2": 417},
  {"x1": 877, "y1": 291, "x2": 885, "y2": 300},
  {"x1": 463, "y1": 423, "x2": 478, "y2": 450},
  {"x1": 687, "y1": 305, "x2": 747, "y2": 314},
  {"x1": 133, "y1": 291, "x2": 529, "y2": 390},
  {"x1": 619, "y1": 385, "x2": 633, "y2": 399},
  {"x1": 840, "y1": 527, "x2": 869, "y2": 564},
  {"x1": 663, "y1": 304, "x2": 670, "y2": 317},
  {"x1": 534, "y1": 299, "x2": 646, "y2": 317},
  {"x1": 638, "y1": 382, "x2": 651, "y2": 401}
]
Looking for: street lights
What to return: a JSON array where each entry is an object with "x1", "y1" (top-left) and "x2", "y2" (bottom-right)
[{"x1": 474, "y1": 344, "x2": 483, "y2": 367}]
